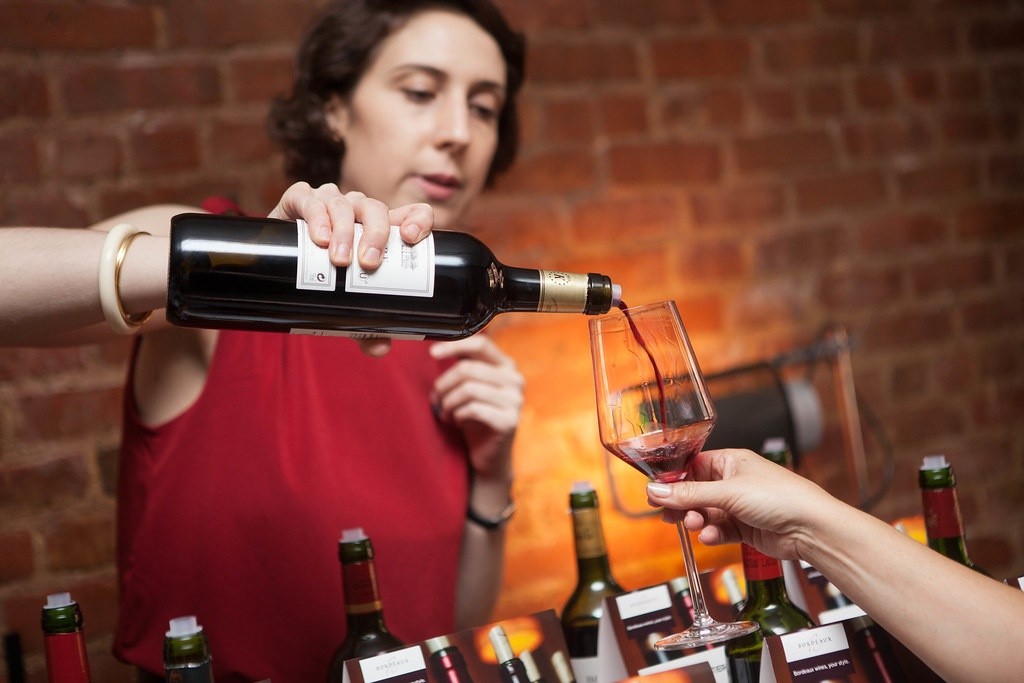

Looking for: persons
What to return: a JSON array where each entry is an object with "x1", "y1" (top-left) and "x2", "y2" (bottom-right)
[
  {"x1": 0, "y1": 0, "x2": 525, "y2": 683},
  {"x1": 645, "y1": 448, "x2": 1024, "y2": 683}
]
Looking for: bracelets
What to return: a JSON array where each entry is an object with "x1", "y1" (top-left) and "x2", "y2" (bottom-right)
[
  {"x1": 114, "y1": 232, "x2": 154, "y2": 326},
  {"x1": 99, "y1": 224, "x2": 151, "y2": 337},
  {"x1": 467, "y1": 497, "x2": 515, "y2": 530}
]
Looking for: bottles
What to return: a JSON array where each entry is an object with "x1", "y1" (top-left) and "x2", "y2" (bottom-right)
[
  {"x1": 488, "y1": 627, "x2": 530, "y2": 683},
  {"x1": 164, "y1": 617, "x2": 214, "y2": 683},
  {"x1": 761, "y1": 439, "x2": 793, "y2": 473},
  {"x1": 166, "y1": 213, "x2": 621, "y2": 341},
  {"x1": 328, "y1": 527, "x2": 405, "y2": 683},
  {"x1": 425, "y1": 636, "x2": 472, "y2": 683},
  {"x1": 42, "y1": 593, "x2": 91, "y2": 683},
  {"x1": 725, "y1": 543, "x2": 814, "y2": 683},
  {"x1": 850, "y1": 616, "x2": 900, "y2": 683},
  {"x1": 917, "y1": 454, "x2": 995, "y2": 579},
  {"x1": 561, "y1": 482, "x2": 625, "y2": 683},
  {"x1": 722, "y1": 570, "x2": 746, "y2": 618},
  {"x1": 669, "y1": 577, "x2": 720, "y2": 654}
]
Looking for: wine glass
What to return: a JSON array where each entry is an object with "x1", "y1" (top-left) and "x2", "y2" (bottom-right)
[{"x1": 589, "y1": 301, "x2": 761, "y2": 652}]
